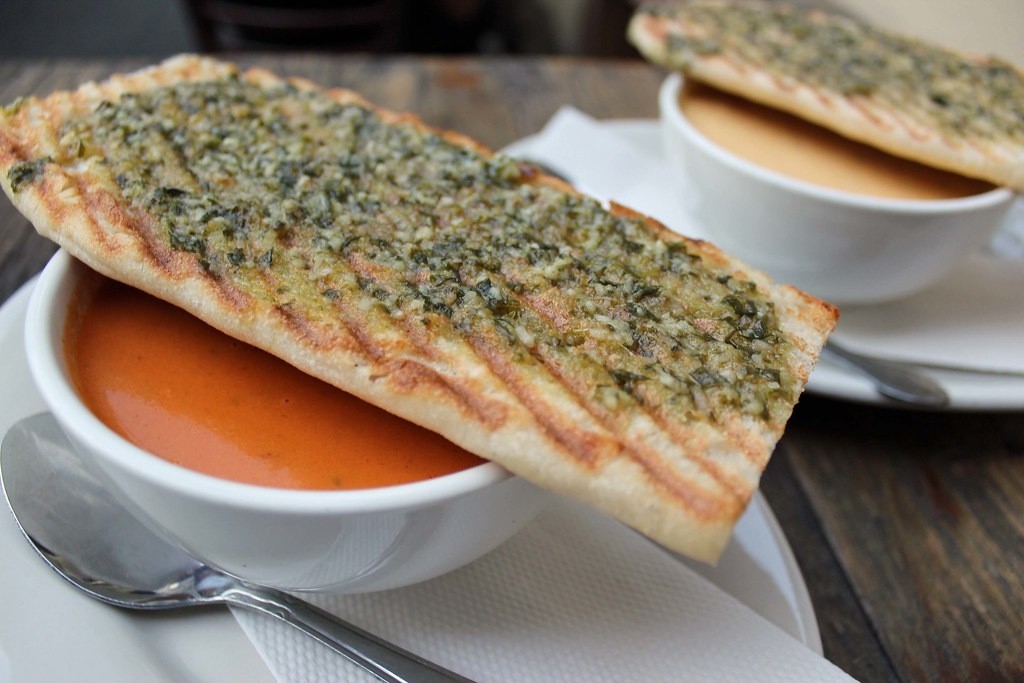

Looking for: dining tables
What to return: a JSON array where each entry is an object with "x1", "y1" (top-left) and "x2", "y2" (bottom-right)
[{"x1": 3, "y1": 53, "x2": 1024, "y2": 683}]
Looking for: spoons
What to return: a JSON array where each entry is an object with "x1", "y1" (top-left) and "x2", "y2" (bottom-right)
[
  {"x1": 819, "y1": 340, "x2": 952, "y2": 410},
  {"x1": 0, "y1": 412, "x2": 482, "y2": 682}
]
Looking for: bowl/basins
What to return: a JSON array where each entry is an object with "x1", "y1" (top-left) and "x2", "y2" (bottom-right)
[
  {"x1": 656, "y1": 68, "x2": 1019, "y2": 314},
  {"x1": 17, "y1": 245, "x2": 545, "y2": 599}
]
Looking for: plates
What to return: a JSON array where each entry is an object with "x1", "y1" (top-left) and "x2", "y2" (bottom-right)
[
  {"x1": 0, "y1": 266, "x2": 822, "y2": 682},
  {"x1": 568, "y1": 123, "x2": 1022, "y2": 410}
]
